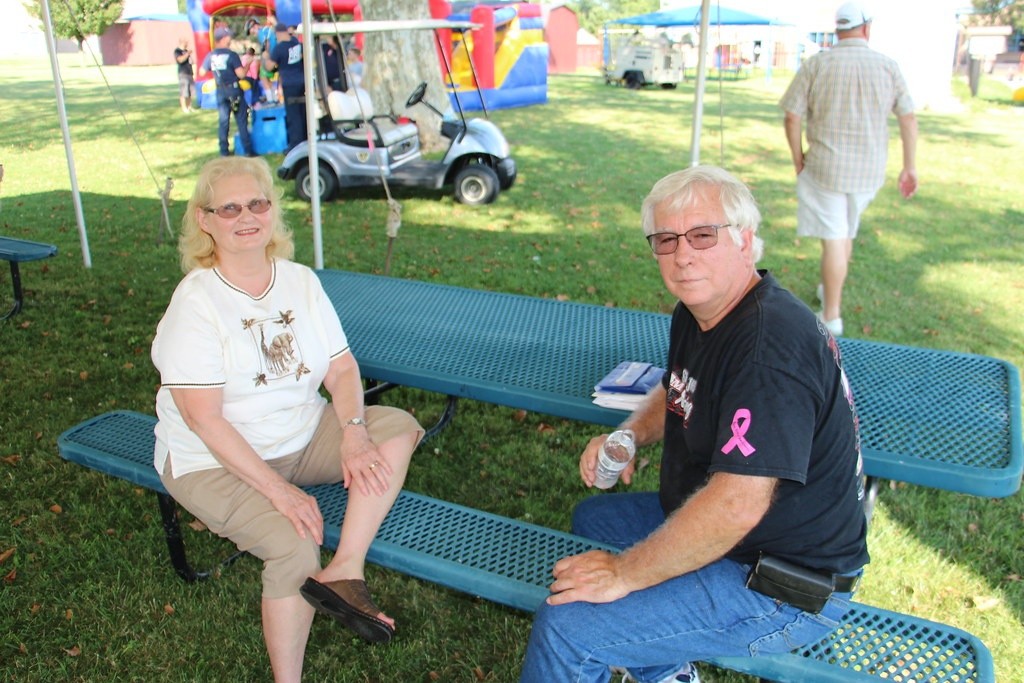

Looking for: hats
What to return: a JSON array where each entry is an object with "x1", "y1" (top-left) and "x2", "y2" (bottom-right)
[
  {"x1": 214, "y1": 27, "x2": 235, "y2": 41},
  {"x1": 276, "y1": 24, "x2": 287, "y2": 31},
  {"x1": 836, "y1": 5, "x2": 871, "y2": 30}
]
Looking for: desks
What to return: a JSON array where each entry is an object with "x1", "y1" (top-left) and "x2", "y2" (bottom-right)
[{"x1": 200, "y1": 269, "x2": 1024, "y2": 591}]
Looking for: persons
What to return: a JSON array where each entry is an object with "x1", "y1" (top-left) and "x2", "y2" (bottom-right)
[
  {"x1": 754, "y1": 45, "x2": 761, "y2": 61},
  {"x1": 244, "y1": 18, "x2": 277, "y2": 106},
  {"x1": 266, "y1": 14, "x2": 308, "y2": 155},
  {"x1": 174, "y1": 38, "x2": 198, "y2": 113},
  {"x1": 520, "y1": 165, "x2": 871, "y2": 683},
  {"x1": 321, "y1": 17, "x2": 367, "y2": 91},
  {"x1": 779, "y1": 1, "x2": 918, "y2": 336},
  {"x1": 150, "y1": 158, "x2": 426, "y2": 683},
  {"x1": 241, "y1": 48, "x2": 262, "y2": 109},
  {"x1": 198, "y1": 28, "x2": 259, "y2": 157}
]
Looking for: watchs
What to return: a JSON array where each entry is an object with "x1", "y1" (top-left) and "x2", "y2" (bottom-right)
[{"x1": 344, "y1": 418, "x2": 365, "y2": 429}]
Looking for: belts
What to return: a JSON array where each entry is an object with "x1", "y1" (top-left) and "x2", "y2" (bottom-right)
[{"x1": 833, "y1": 574, "x2": 859, "y2": 593}]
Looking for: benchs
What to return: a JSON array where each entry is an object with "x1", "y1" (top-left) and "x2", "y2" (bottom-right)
[
  {"x1": 58, "y1": 409, "x2": 994, "y2": 683},
  {"x1": 0, "y1": 236, "x2": 57, "y2": 313},
  {"x1": 328, "y1": 87, "x2": 418, "y2": 147}
]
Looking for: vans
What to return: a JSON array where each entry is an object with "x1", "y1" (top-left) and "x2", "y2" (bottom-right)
[{"x1": 610, "y1": 46, "x2": 683, "y2": 90}]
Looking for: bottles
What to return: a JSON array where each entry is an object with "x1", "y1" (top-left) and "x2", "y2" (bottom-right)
[{"x1": 592, "y1": 429, "x2": 637, "y2": 490}]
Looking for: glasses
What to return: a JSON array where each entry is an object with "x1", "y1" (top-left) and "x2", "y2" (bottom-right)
[
  {"x1": 200, "y1": 199, "x2": 271, "y2": 218},
  {"x1": 646, "y1": 224, "x2": 731, "y2": 255}
]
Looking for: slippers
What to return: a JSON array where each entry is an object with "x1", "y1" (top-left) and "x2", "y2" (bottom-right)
[{"x1": 299, "y1": 577, "x2": 393, "y2": 644}]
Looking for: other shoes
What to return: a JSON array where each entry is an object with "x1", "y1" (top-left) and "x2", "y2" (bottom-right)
[
  {"x1": 816, "y1": 284, "x2": 824, "y2": 308},
  {"x1": 621, "y1": 663, "x2": 700, "y2": 683},
  {"x1": 814, "y1": 312, "x2": 843, "y2": 335}
]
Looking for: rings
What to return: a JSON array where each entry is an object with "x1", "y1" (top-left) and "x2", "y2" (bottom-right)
[{"x1": 370, "y1": 463, "x2": 376, "y2": 469}]
